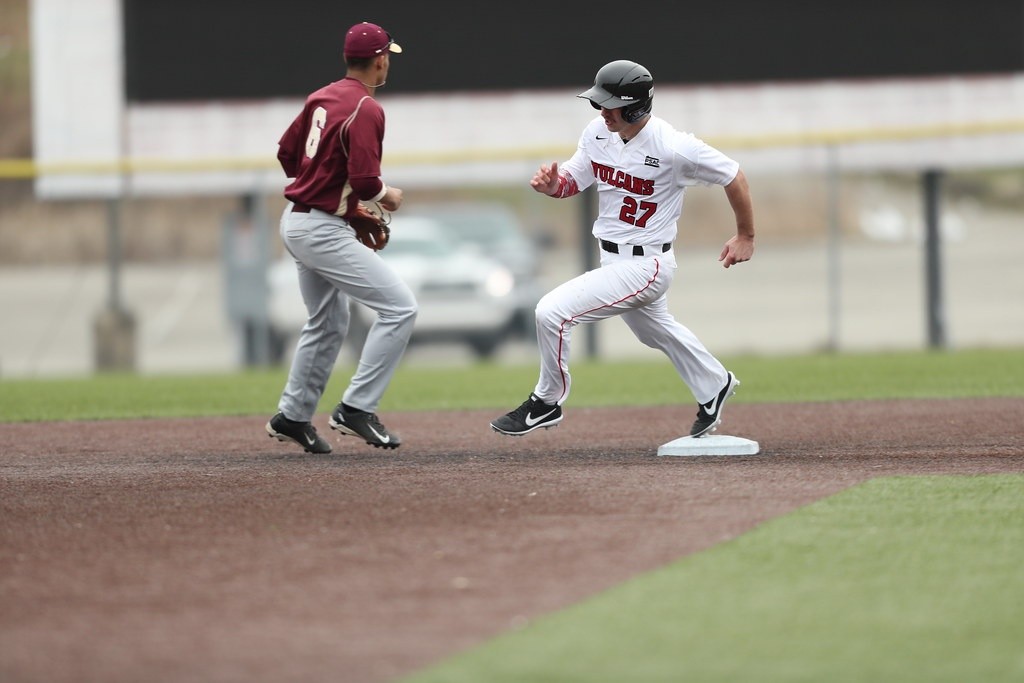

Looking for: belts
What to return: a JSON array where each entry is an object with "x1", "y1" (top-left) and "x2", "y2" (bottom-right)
[
  {"x1": 600, "y1": 239, "x2": 671, "y2": 256},
  {"x1": 292, "y1": 203, "x2": 311, "y2": 213}
]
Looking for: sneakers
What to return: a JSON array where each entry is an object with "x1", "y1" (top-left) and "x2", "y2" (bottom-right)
[
  {"x1": 328, "y1": 401, "x2": 401, "y2": 450},
  {"x1": 489, "y1": 392, "x2": 565, "y2": 437},
  {"x1": 266, "y1": 410, "x2": 332, "y2": 453},
  {"x1": 690, "y1": 370, "x2": 741, "y2": 437}
]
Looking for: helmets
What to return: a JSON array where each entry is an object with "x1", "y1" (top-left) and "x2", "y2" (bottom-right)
[{"x1": 576, "y1": 60, "x2": 654, "y2": 123}]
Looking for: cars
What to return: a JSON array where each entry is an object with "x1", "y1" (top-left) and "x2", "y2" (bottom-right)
[{"x1": 250, "y1": 207, "x2": 557, "y2": 360}]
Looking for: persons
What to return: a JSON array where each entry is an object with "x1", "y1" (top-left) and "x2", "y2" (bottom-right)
[
  {"x1": 491, "y1": 60, "x2": 754, "y2": 440},
  {"x1": 265, "y1": 22, "x2": 418, "y2": 454}
]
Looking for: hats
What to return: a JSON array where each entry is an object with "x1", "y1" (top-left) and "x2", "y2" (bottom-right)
[{"x1": 344, "y1": 22, "x2": 402, "y2": 60}]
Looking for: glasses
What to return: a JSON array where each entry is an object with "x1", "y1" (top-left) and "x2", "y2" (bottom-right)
[{"x1": 375, "y1": 31, "x2": 394, "y2": 55}]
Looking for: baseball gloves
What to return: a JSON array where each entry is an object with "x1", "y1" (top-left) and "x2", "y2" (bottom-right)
[{"x1": 340, "y1": 202, "x2": 390, "y2": 250}]
